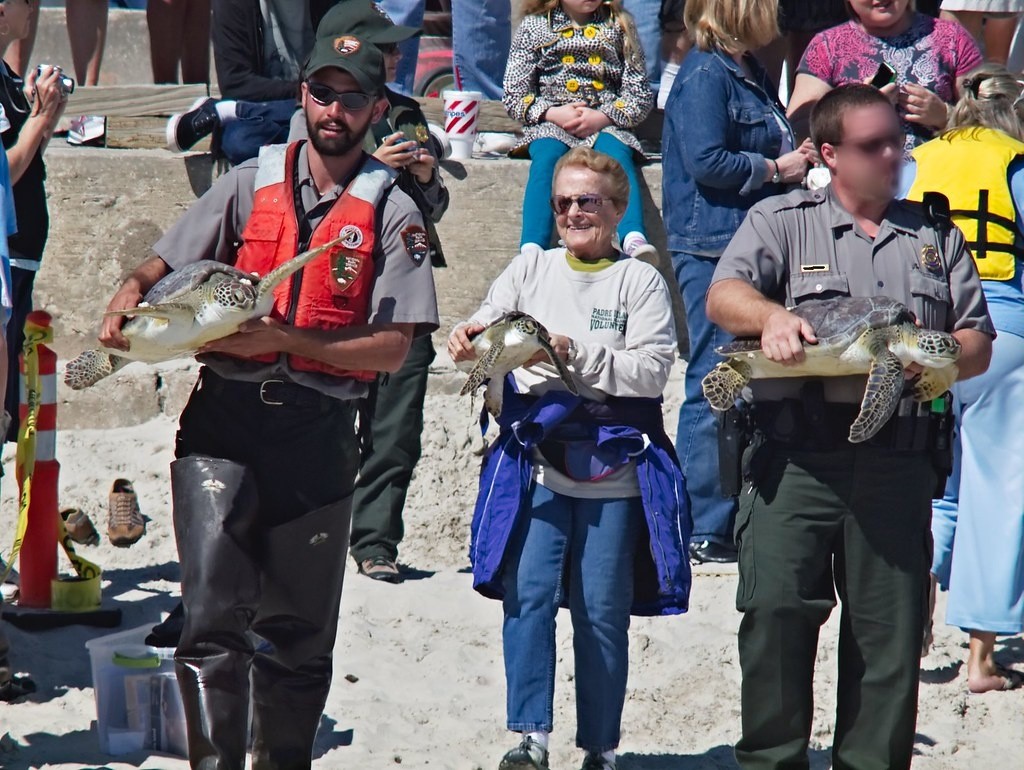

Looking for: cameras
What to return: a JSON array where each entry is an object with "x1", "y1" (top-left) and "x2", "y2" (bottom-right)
[
  {"x1": 35, "y1": 64, "x2": 74, "y2": 94},
  {"x1": 871, "y1": 61, "x2": 897, "y2": 90}
]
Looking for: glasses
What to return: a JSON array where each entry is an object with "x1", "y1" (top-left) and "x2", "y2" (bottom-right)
[
  {"x1": 375, "y1": 40, "x2": 398, "y2": 54},
  {"x1": 825, "y1": 133, "x2": 907, "y2": 156},
  {"x1": 550, "y1": 192, "x2": 613, "y2": 213},
  {"x1": 304, "y1": 77, "x2": 378, "y2": 113}
]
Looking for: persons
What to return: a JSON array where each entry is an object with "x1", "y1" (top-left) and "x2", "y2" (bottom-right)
[
  {"x1": 318, "y1": 0, "x2": 451, "y2": 581},
  {"x1": 704, "y1": 84, "x2": 997, "y2": 770},
  {"x1": 100, "y1": 36, "x2": 441, "y2": 770},
  {"x1": 898, "y1": 69, "x2": 1024, "y2": 693},
  {"x1": 0, "y1": 0, "x2": 67, "y2": 478},
  {"x1": 503, "y1": 0, "x2": 660, "y2": 269},
  {"x1": 658, "y1": 0, "x2": 1024, "y2": 151},
  {"x1": 663, "y1": 0, "x2": 824, "y2": 560},
  {"x1": 63, "y1": 0, "x2": 666, "y2": 96},
  {"x1": 448, "y1": 149, "x2": 692, "y2": 770}
]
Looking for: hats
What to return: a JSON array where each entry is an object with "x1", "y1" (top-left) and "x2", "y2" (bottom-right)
[
  {"x1": 317, "y1": 0, "x2": 424, "y2": 50},
  {"x1": 304, "y1": 34, "x2": 385, "y2": 95}
]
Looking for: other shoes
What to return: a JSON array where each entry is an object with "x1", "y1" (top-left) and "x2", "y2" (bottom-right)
[{"x1": 688, "y1": 539, "x2": 736, "y2": 562}]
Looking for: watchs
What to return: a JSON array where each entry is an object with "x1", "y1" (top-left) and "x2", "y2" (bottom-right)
[{"x1": 770, "y1": 158, "x2": 780, "y2": 184}]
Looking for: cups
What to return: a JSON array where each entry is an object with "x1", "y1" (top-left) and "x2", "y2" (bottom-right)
[{"x1": 441, "y1": 90, "x2": 482, "y2": 160}]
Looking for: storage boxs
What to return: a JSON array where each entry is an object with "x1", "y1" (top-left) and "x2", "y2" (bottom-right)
[{"x1": 83, "y1": 623, "x2": 195, "y2": 760}]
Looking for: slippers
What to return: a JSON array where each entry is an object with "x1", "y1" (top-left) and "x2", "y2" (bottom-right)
[
  {"x1": 921, "y1": 620, "x2": 934, "y2": 658},
  {"x1": 965, "y1": 664, "x2": 1024, "y2": 692}
]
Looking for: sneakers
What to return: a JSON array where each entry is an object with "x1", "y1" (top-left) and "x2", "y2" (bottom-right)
[
  {"x1": 499, "y1": 734, "x2": 549, "y2": 769},
  {"x1": 106, "y1": 477, "x2": 146, "y2": 544},
  {"x1": 581, "y1": 748, "x2": 616, "y2": 770},
  {"x1": 166, "y1": 95, "x2": 222, "y2": 153},
  {"x1": 362, "y1": 555, "x2": 398, "y2": 583},
  {"x1": 59, "y1": 506, "x2": 97, "y2": 544}
]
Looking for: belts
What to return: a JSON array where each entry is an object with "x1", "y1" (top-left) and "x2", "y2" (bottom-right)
[{"x1": 199, "y1": 369, "x2": 354, "y2": 408}]
